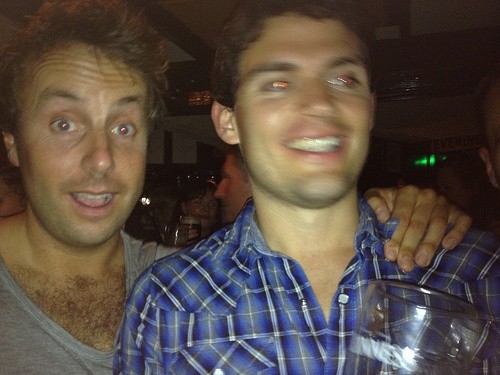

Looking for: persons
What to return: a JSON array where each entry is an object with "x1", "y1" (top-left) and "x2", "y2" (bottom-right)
[
  {"x1": 0, "y1": 147, "x2": 253, "y2": 248},
  {"x1": 0, "y1": 0, "x2": 472, "y2": 375},
  {"x1": 111, "y1": 0, "x2": 500, "y2": 375},
  {"x1": 479, "y1": 75, "x2": 500, "y2": 191}
]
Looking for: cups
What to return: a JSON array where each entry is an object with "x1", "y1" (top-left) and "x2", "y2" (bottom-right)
[
  {"x1": 164, "y1": 224, "x2": 187, "y2": 249},
  {"x1": 182, "y1": 215, "x2": 201, "y2": 242},
  {"x1": 343, "y1": 279, "x2": 480, "y2": 375}
]
